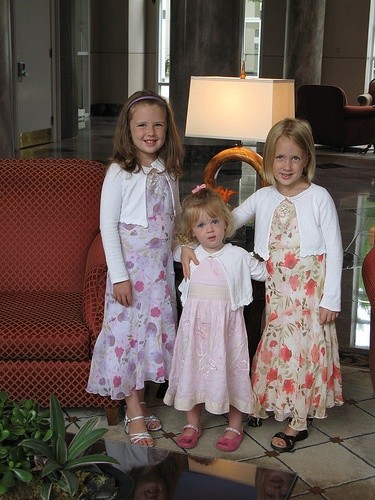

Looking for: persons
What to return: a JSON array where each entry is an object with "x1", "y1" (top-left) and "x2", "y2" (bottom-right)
[
  {"x1": 171, "y1": 182, "x2": 268, "y2": 451},
  {"x1": 86, "y1": 90, "x2": 184, "y2": 447},
  {"x1": 173, "y1": 118, "x2": 344, "y2": 450}
]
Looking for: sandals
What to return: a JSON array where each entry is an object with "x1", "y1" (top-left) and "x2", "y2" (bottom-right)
[
  {"x1": 177, "y1": 424, "x2": 202, "y2": 449},
  {"x1": 124, "y1": 412, "x2": 155, "y2": 447},
  {"x1": 125, "y1": 402, "x2": 162, "y2": 431},
  {"x1": 287, "y1": 417, "x2": 313, "y2": 428},
  {"x1": 271, "y1": 430, "x2": 309, "y2": 452},
  {"x1": 215, "y1": 426, "x2": 245, "y2": 452}
]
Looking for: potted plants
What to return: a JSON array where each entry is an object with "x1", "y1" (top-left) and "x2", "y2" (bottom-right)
[{"x1": 0, "y1": 391, "x2": 134, "y2": 500}]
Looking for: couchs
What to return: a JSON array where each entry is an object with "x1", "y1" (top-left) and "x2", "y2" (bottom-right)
[
  {"x1": 298, "y1": 79, "x2": 375, "y2": 152},
  {"x1": 0, "y1": 158, "x2": 121, "y2": 425}
]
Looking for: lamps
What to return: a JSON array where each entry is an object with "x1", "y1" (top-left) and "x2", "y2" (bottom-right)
[{"x1": 185, "y1": 61, "x2": 294, "y2": 207}]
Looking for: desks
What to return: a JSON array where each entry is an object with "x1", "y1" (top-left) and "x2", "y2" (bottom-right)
[{"x1": 105, "y1": 439, "x2": 297, "y2": 500}]
